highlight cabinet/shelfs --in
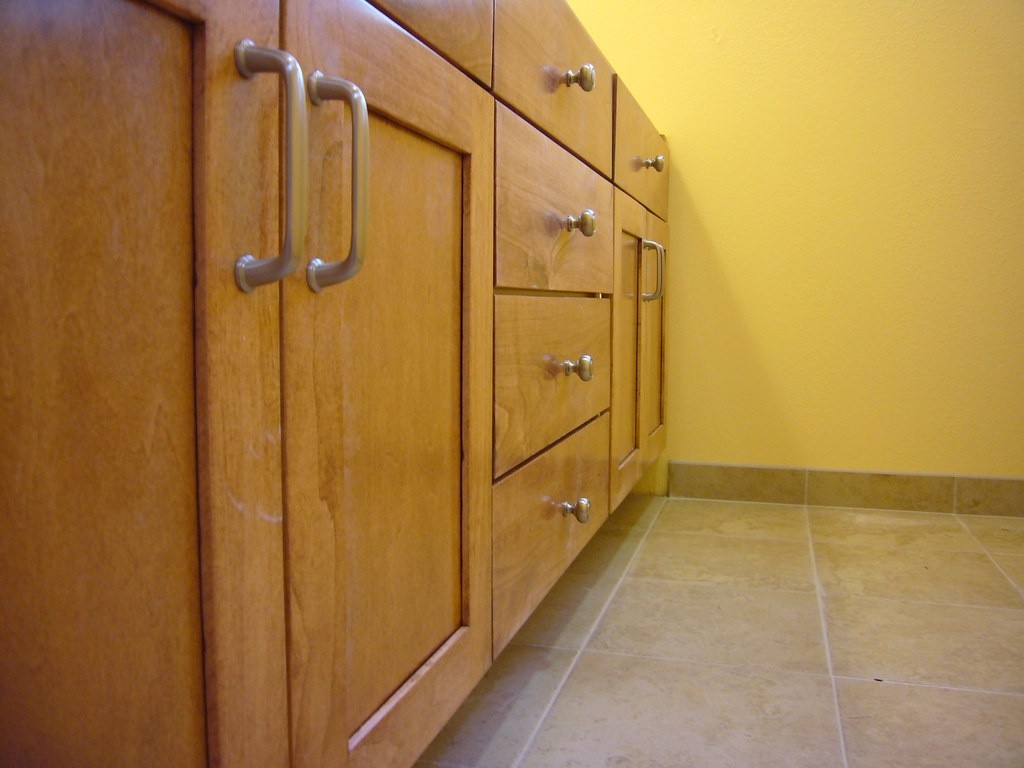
[0,0,496,768]
[615,73,670,487]
[492,0,615,666]
